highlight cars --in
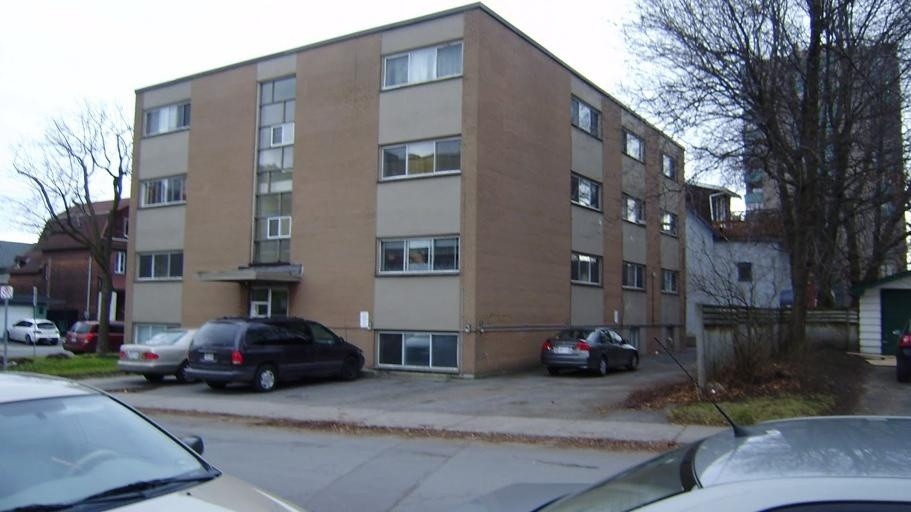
[7,318,60,346]
[540,326,639,376]
[62,320,123,355]
[116,328,201,383]
[465,413,911,512]
[891,318,911,383]
[0,369,305,512]
[183,317,364,393]
